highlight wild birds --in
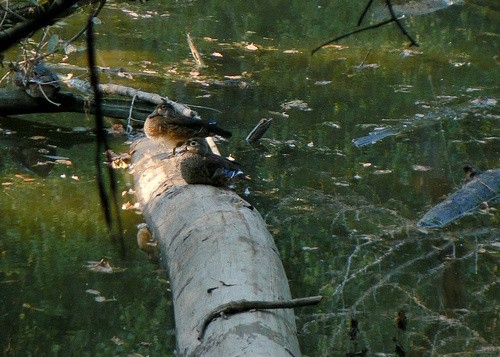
[177,136,246,187]
[144,102,233,160]
